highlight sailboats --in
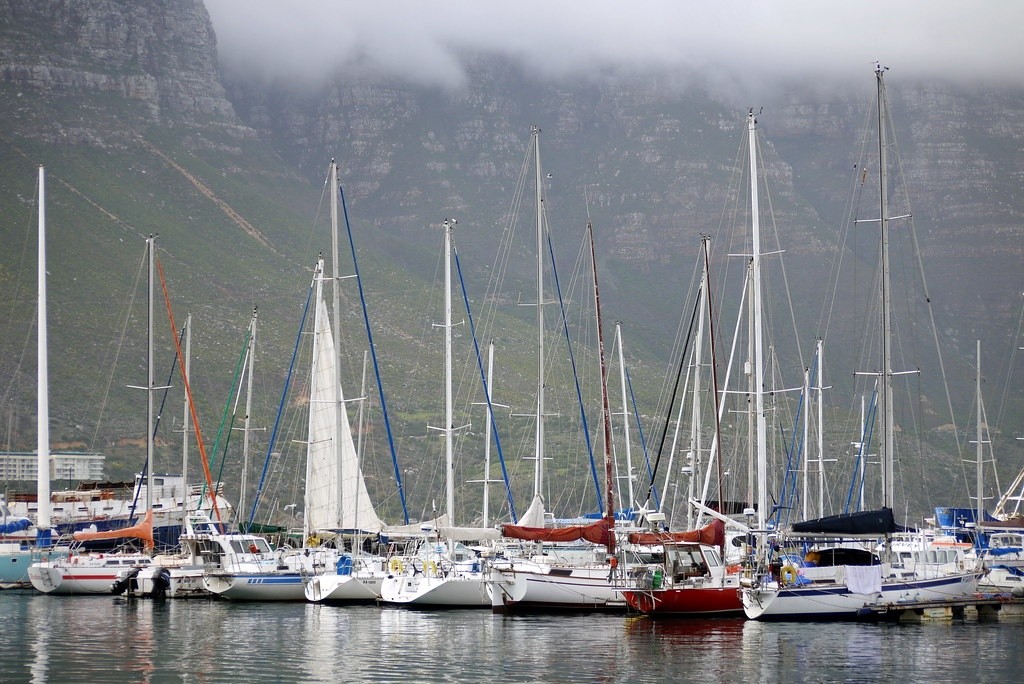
[1,52,1024,622]
